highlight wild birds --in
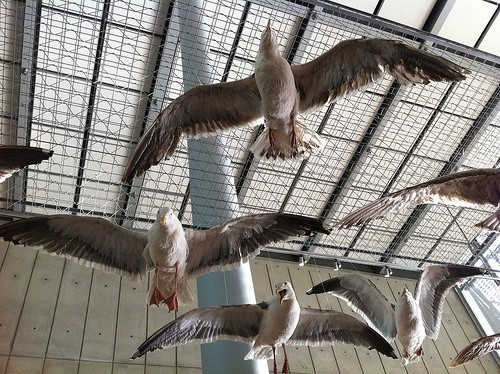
[305,264,490,366]
[128,280,400,361]
[0,207,331,314]
[449,332,500,368]
[0,144,54,185]
[331,167,500,233]
[120,18,472,184]
